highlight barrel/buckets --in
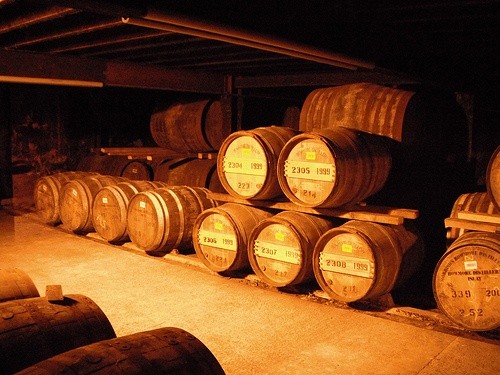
[0,266,228,375]
[31,83,500,332]
